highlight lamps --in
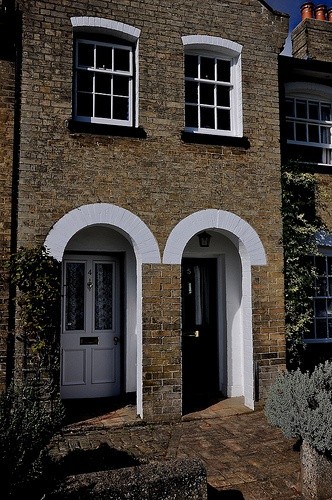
[196,230,213,247]
